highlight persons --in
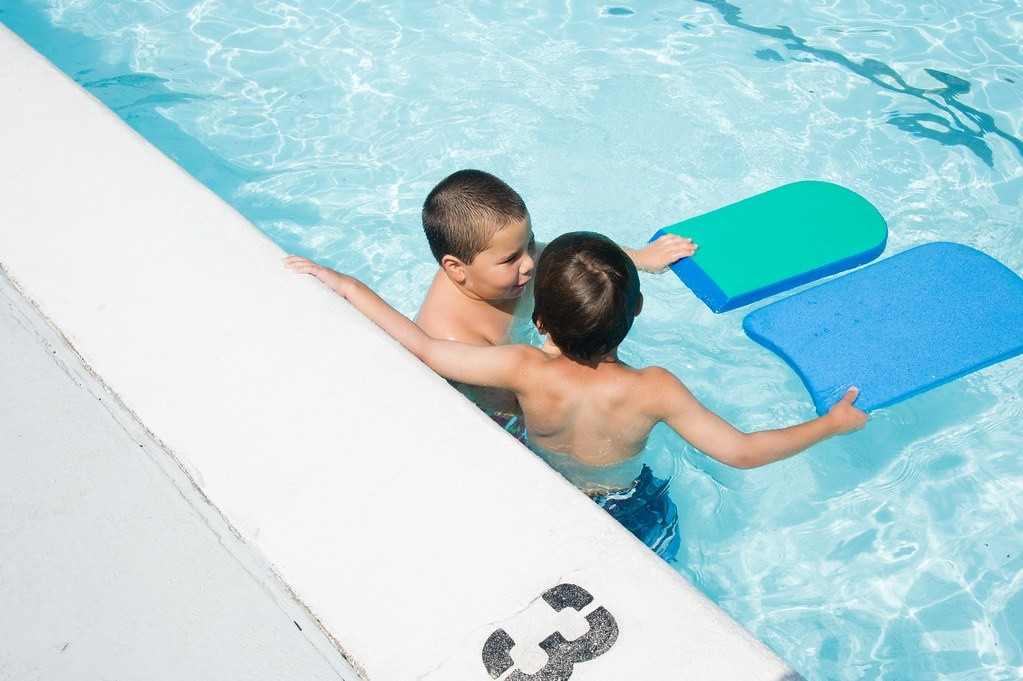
[284,230,869,469]
[411,169,699,348]
